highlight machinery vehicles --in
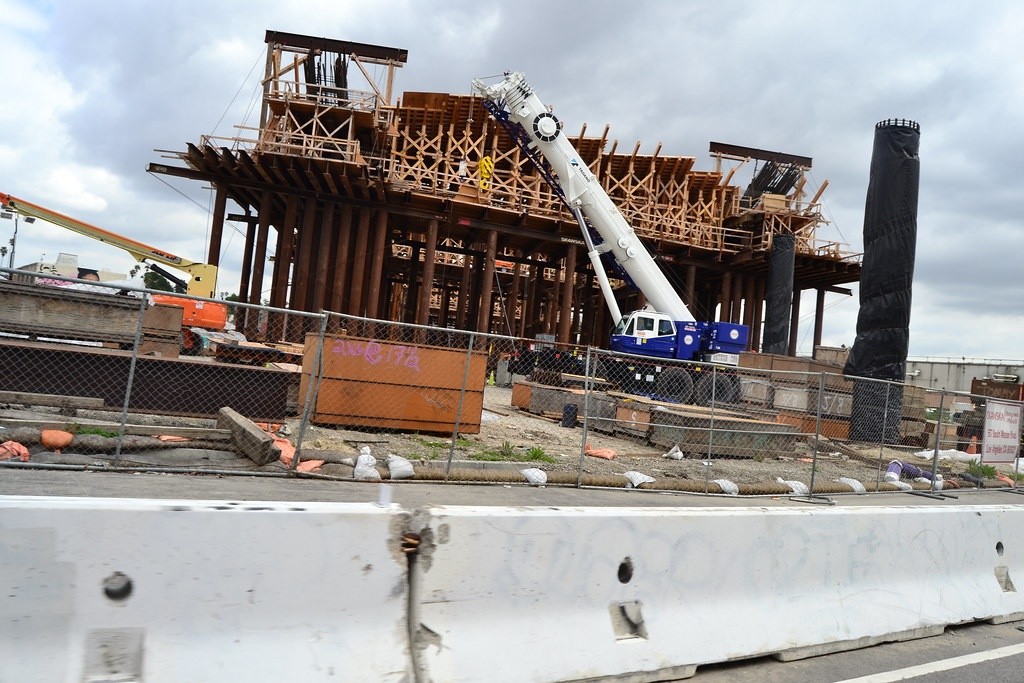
[1,189,226,358]
[468,67,749,402]
